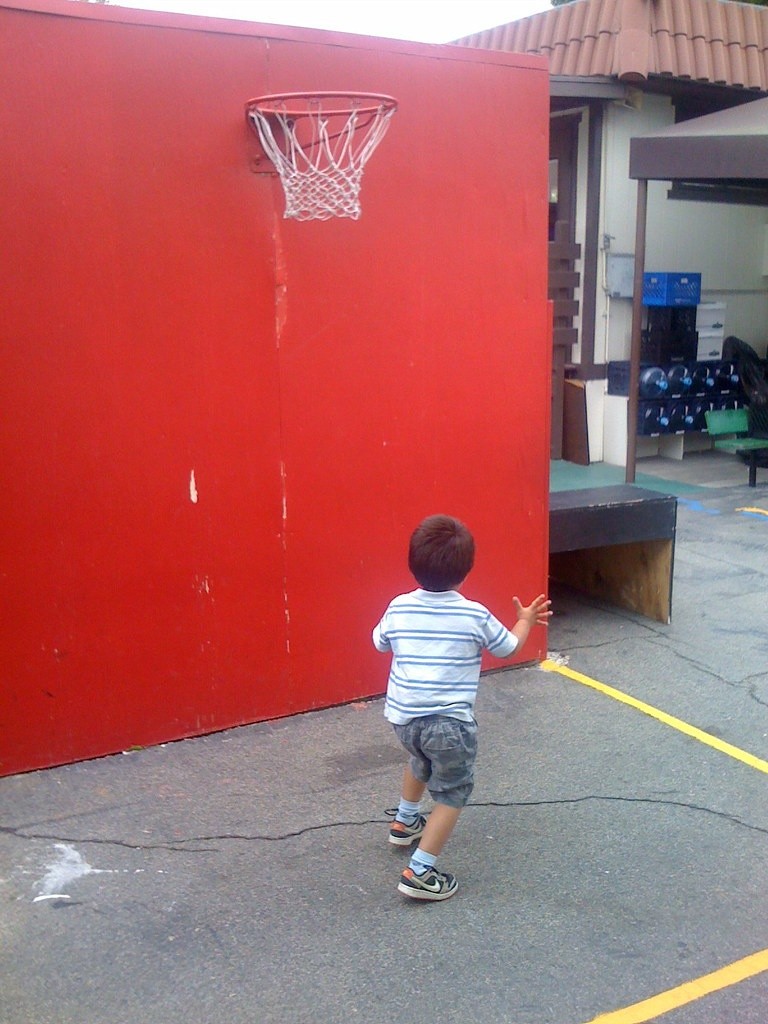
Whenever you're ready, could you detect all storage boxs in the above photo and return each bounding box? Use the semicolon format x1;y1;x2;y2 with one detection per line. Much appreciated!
642;272;728;367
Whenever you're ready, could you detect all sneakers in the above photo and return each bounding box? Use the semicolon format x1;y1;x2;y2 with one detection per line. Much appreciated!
384;808;428;846
397;865;459;900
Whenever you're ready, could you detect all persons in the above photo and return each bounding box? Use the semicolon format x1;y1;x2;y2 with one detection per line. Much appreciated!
371;514;552;901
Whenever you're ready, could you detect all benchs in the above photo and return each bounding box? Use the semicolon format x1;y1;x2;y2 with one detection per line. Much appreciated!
705;408;768;488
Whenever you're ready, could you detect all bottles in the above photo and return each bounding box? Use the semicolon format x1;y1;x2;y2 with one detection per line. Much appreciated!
607;360;669;400
690;396;742;433
661;363;693;397
708;361;739;390
666;399;695;433
638;400;671;435
685;363;716;392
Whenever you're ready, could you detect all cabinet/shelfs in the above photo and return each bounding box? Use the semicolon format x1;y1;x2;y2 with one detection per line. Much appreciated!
603;391;737;467
